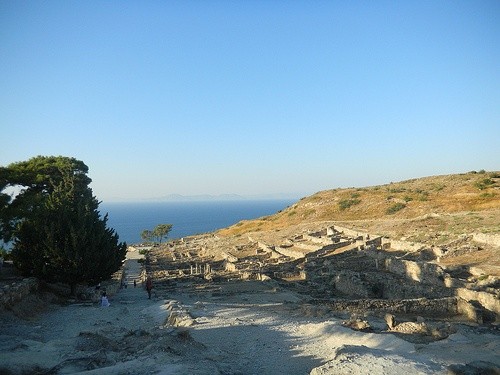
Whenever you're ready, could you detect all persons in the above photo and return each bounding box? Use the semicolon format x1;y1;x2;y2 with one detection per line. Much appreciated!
124;279;128;288
134;279;137;288
146;275;152;299
